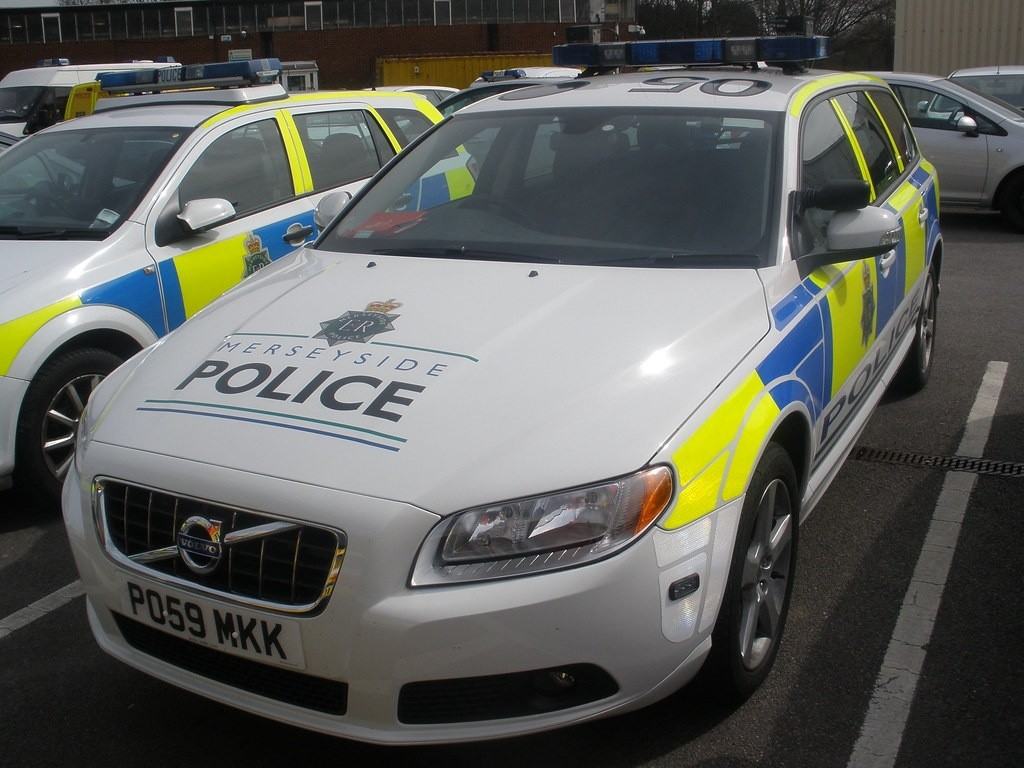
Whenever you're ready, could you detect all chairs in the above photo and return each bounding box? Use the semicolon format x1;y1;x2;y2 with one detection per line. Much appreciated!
317;132;365;184
668;149;747;263
143;146;170;190
539;140;643;245
736;128;771;199
633;120;722;200
217;136;274;203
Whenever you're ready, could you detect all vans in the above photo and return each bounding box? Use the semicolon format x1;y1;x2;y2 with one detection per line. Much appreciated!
0;55;183;154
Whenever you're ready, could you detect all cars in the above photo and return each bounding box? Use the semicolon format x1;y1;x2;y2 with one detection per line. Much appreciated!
853;71;1024;235
59;35;942;749
305;86;460;146
916;65;1024;120
0;58;490;524
463;67;583;108
